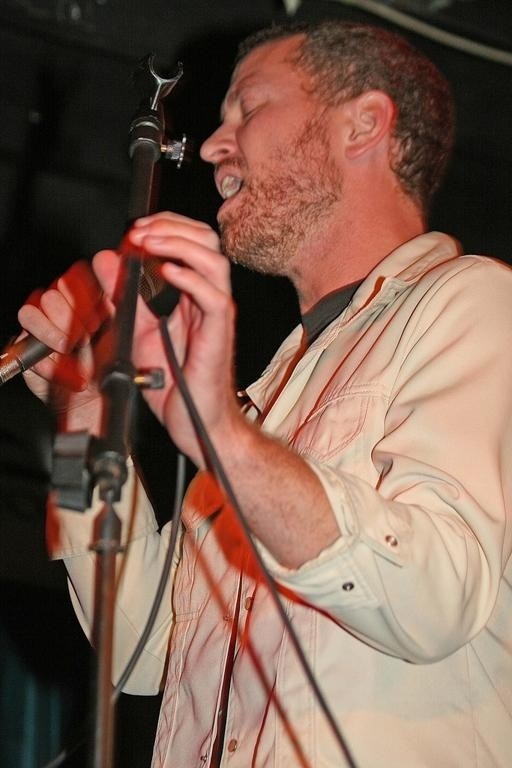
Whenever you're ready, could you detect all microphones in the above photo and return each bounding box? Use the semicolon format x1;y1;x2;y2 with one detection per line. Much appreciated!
1;260;176;388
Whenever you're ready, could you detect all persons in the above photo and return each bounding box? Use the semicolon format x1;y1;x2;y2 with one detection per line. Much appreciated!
13;13;512;768
